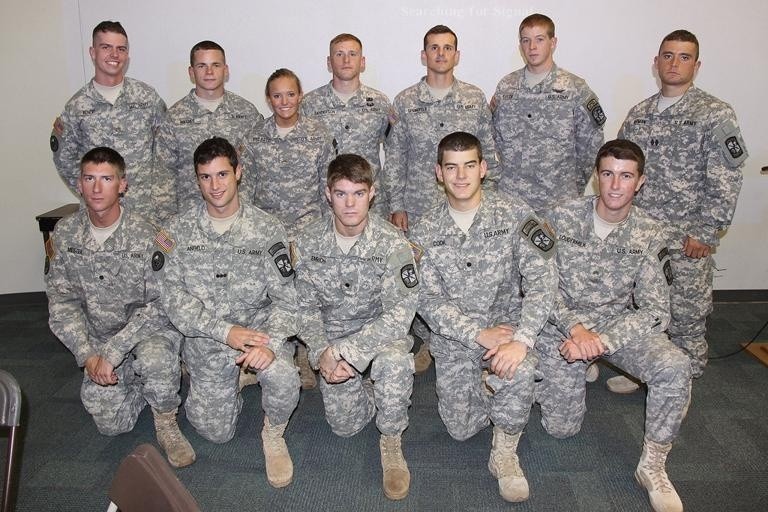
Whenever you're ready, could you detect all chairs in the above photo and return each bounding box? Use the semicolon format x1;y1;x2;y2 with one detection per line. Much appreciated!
0;367;26;511
105;442;201;512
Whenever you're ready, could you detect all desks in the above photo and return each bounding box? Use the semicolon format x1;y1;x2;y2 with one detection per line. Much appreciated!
35;202;81;254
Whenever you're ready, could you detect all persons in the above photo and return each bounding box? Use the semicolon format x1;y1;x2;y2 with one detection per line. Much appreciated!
42;146;200;471
532;137;698;510
151;39;268;395
294;149;421;501
382;23;501;381
604;25;751;397
237;66;338;395
48;18;184;380
152;137;301;490
407;128;562;504
489;11;610;387
294;33;408;234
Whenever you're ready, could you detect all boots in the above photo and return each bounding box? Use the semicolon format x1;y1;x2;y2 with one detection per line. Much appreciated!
295;344;318;390
150;406;197;469
487;426;531;503
260;414;295;489
379;433;411;502
632;436;685;512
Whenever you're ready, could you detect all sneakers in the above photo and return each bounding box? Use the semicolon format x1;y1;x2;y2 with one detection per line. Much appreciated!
413;343;429;376
238;367;259;392
584;363;600;384
606;374;644;395
